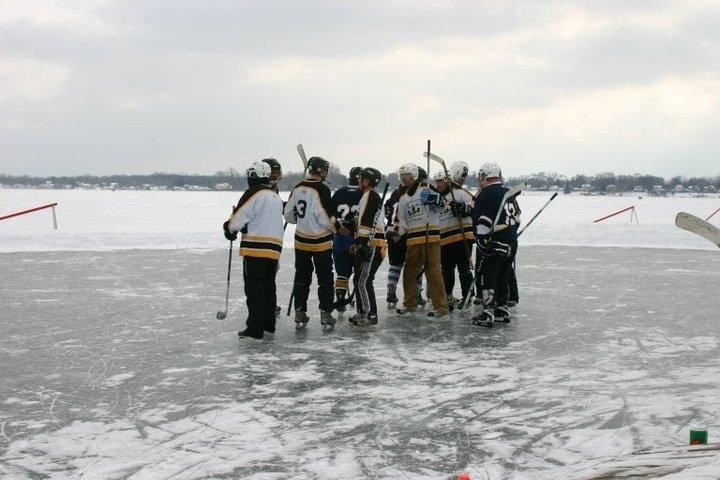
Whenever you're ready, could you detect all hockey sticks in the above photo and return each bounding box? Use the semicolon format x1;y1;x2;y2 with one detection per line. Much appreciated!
283;144;308;232
462;182;527;312
675;211;720;247
416;140;430;278
423;151;474;270
319;182;389;308
217;206;236;319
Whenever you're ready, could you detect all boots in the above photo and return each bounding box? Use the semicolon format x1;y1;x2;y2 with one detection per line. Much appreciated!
386;284;398;303
472;297;496;323
495;296;510;317
395;285;518;309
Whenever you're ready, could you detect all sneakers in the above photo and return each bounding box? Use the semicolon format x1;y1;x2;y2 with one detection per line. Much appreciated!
396;308;415;314
238;307;281;340
348;311;378;326
321;310;337;324
427;308;446;317
295;310;309;323
337;294;346;312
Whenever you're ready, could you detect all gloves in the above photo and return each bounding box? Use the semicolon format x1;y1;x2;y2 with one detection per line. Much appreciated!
449;200;473;217
420;188;443;208
223;220;239;241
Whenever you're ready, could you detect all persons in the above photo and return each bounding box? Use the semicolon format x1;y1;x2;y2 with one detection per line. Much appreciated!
222;157;522;339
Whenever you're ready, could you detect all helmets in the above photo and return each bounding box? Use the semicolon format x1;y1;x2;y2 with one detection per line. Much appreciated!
246;158;282;188
398;162;427;182
308;157;329;175
433;168;454;182
348;166;382;188
476;161;502;183
